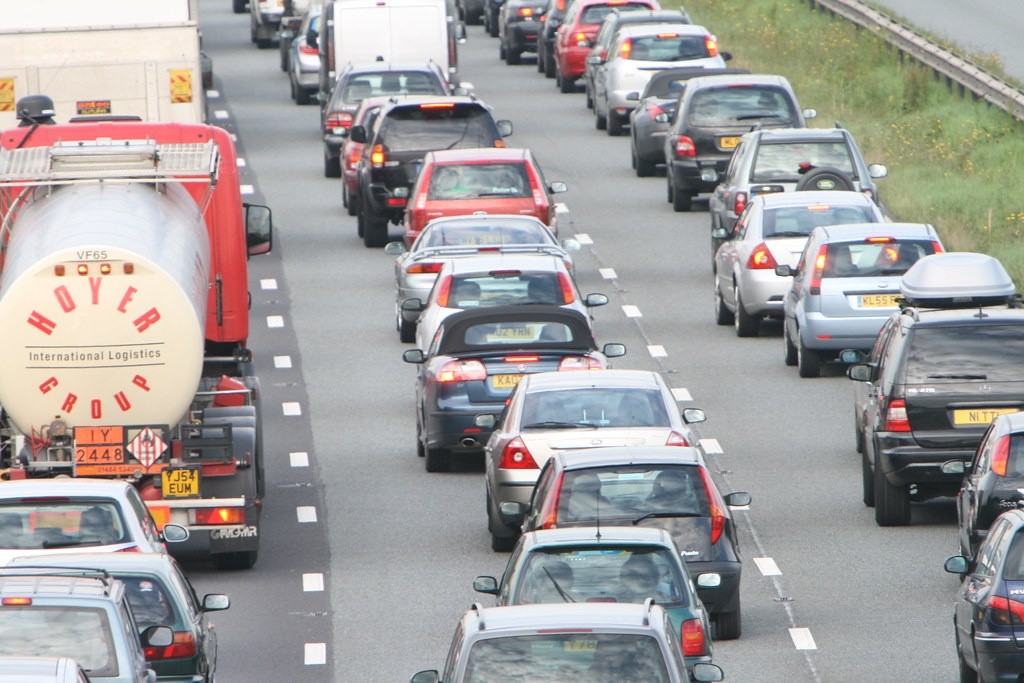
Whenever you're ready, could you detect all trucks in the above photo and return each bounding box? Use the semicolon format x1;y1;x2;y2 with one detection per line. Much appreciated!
316;0;456;122
1;94;275;575
1;0;214;125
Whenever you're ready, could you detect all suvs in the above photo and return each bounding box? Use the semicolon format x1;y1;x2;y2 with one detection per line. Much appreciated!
350;96;514;248
576;6;695;107
839;251;1024;526
709;119;888;244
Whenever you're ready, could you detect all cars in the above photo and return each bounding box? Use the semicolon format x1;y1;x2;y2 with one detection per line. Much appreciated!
454;0;661;93
586;25;734;135
0;564;157;683
774;223;947;374
943;500;1024;683
484;370;706;553
415;251;608;376
316;61;476;178
474;526;714;681
410;601;725;683
655;73;819;213
332;95;477;223
711;189;888;346
4;551;230;683
626;65;753;177
0;477;190;553
523;442;752;643
938;411;1024;584
402;305;627;474
392;146;569;249
385;214;582;346
0;656;92;682
232;0;323;105
